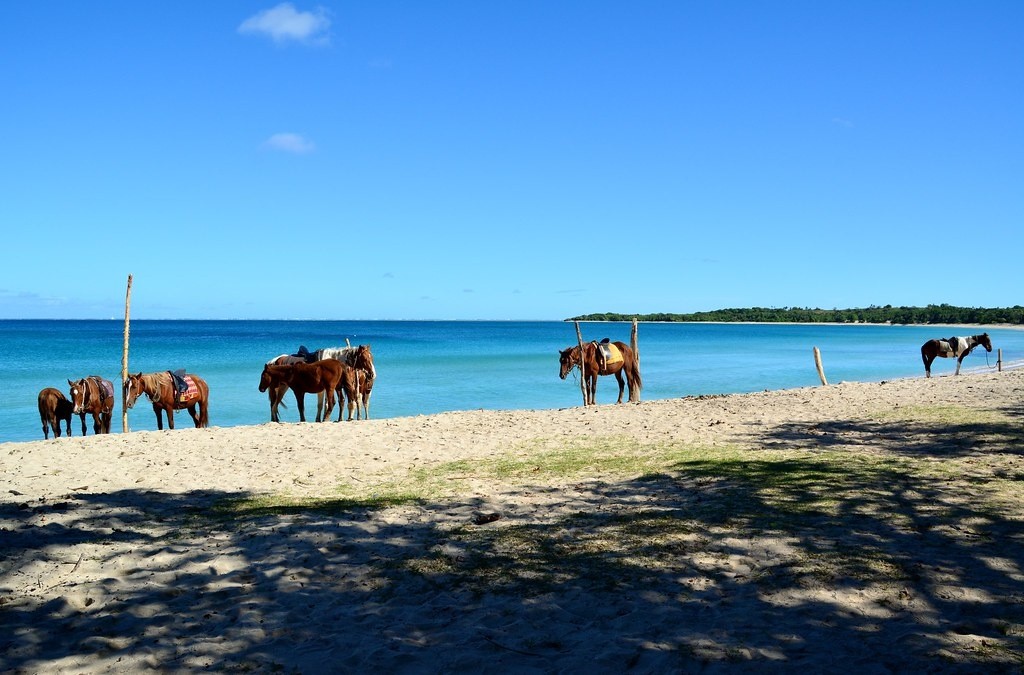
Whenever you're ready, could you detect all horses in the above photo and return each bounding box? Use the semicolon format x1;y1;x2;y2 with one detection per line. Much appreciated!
125;371;209;430
259;345;376;423
38;388;73;439
559;342;642;405
68;376;114;436
921;333;992;378
